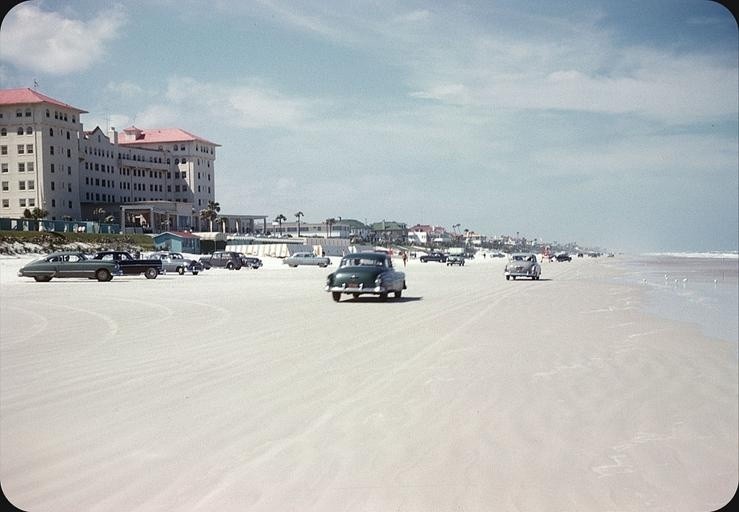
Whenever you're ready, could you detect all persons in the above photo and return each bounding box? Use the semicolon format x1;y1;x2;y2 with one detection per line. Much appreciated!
402;251;408;266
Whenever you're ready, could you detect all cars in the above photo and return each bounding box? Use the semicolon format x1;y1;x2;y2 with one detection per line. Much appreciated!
150;250;263;282
419;251;465;267
504;252;542;280
323;252;407;301
19;251;162;282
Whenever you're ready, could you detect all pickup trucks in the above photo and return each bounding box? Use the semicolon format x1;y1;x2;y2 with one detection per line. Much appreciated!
283;252;330;267
